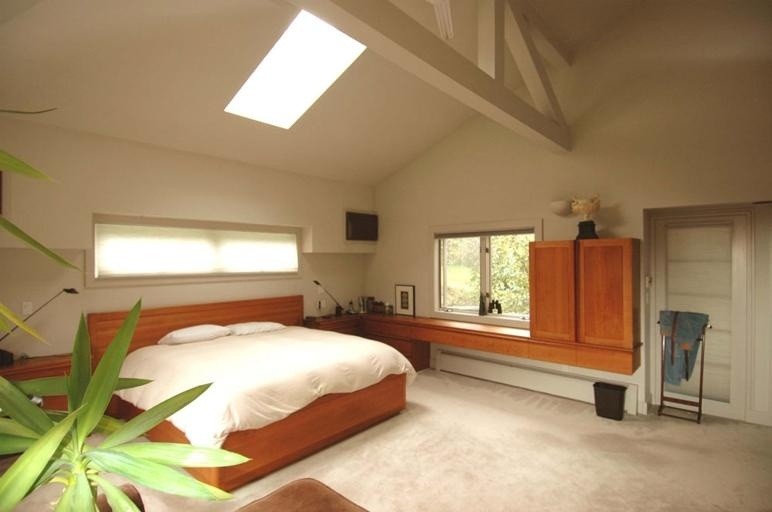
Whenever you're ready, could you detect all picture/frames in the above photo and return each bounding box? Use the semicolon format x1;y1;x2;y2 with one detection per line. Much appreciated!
394;284;416;316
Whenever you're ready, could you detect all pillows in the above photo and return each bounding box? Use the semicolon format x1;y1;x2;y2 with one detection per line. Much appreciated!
159;320;285;345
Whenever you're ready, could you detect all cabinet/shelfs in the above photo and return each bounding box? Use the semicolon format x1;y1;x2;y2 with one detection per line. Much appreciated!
529;237;642;349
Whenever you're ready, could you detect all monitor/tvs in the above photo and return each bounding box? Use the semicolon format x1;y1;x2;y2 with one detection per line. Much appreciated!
345;211;379;242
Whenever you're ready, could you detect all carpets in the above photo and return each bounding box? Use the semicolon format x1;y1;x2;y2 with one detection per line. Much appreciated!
235;478;367;512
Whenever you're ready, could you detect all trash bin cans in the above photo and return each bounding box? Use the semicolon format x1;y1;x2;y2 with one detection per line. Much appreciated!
593;382;628;421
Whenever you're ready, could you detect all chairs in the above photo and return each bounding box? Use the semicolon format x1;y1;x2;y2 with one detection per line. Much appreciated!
95;483;145;512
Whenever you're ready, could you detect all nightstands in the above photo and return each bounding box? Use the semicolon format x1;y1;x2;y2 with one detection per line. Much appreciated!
305;313;366;337
0;354;73;411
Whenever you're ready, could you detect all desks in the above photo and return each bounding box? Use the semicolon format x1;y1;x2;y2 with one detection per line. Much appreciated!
363;313;644;375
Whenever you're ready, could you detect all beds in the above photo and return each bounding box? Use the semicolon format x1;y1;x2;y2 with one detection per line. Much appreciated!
87;293;407;492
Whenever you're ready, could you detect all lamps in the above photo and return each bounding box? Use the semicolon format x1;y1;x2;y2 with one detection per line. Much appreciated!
312;279;345;316
0;288;79;365
